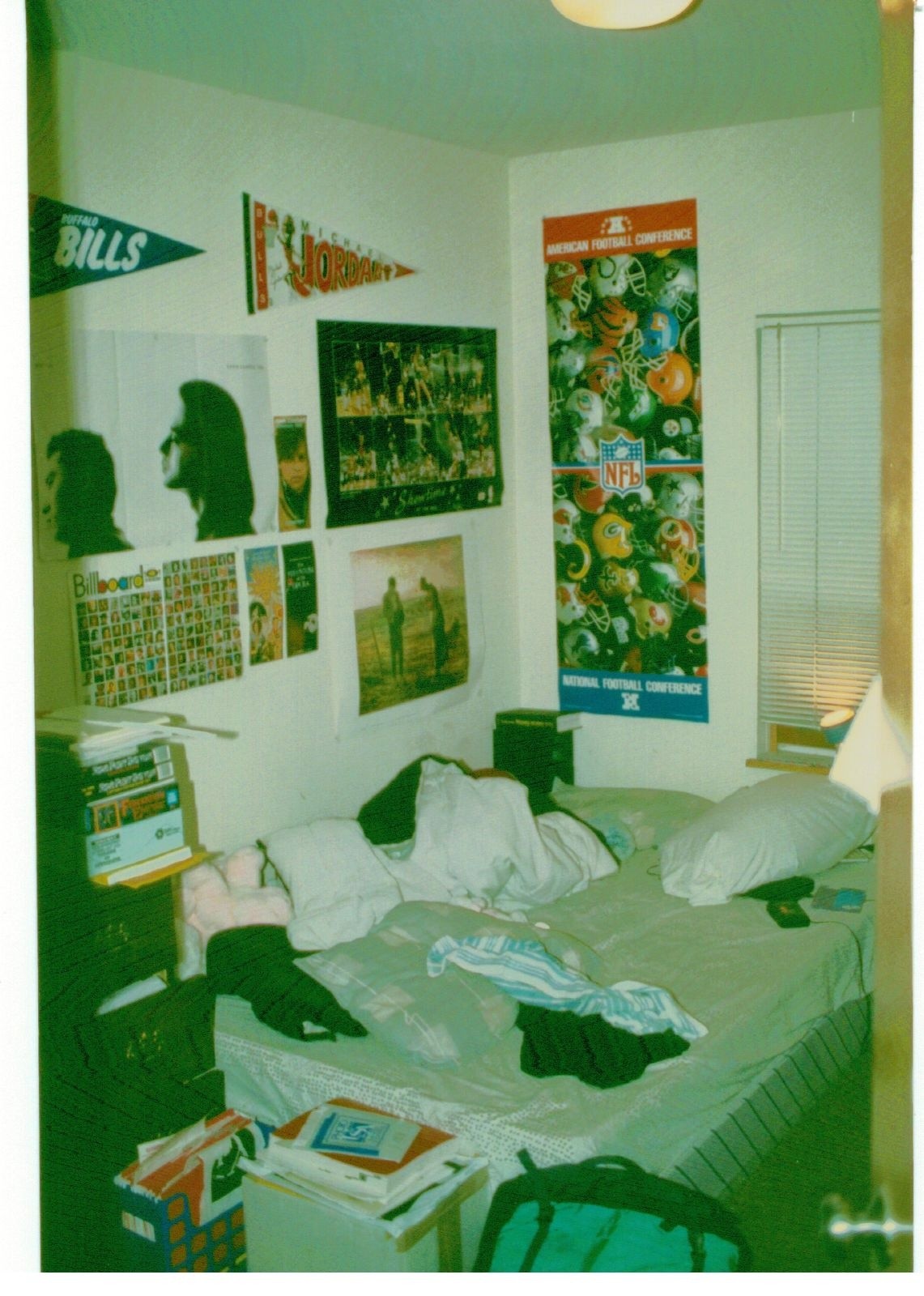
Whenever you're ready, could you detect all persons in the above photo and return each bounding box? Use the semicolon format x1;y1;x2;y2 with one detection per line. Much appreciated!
381;577;409;680
334;339;497;497
417;575;452;678
273;419;316;534
66;549;245;708
158;378;258;541
38;428;133;558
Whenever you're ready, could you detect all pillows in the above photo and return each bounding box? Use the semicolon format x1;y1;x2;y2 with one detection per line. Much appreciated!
295;898;602;1077
658;771;877;908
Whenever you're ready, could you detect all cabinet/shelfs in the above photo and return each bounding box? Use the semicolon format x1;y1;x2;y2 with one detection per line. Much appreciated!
40;869;225;1272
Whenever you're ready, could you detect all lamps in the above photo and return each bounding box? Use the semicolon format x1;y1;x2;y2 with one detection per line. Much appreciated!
819;706;854;748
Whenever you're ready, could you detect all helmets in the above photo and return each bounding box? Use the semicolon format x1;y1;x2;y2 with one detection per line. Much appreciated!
546;247;708;680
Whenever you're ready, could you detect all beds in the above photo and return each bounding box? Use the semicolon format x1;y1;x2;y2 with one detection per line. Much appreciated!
176;778;880;1219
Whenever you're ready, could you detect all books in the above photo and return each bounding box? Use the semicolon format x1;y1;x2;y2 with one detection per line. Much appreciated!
114;1092;494;1274
36;700;192;890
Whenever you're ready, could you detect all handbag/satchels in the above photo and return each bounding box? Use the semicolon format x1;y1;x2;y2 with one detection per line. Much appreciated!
472;1148;751;1272
